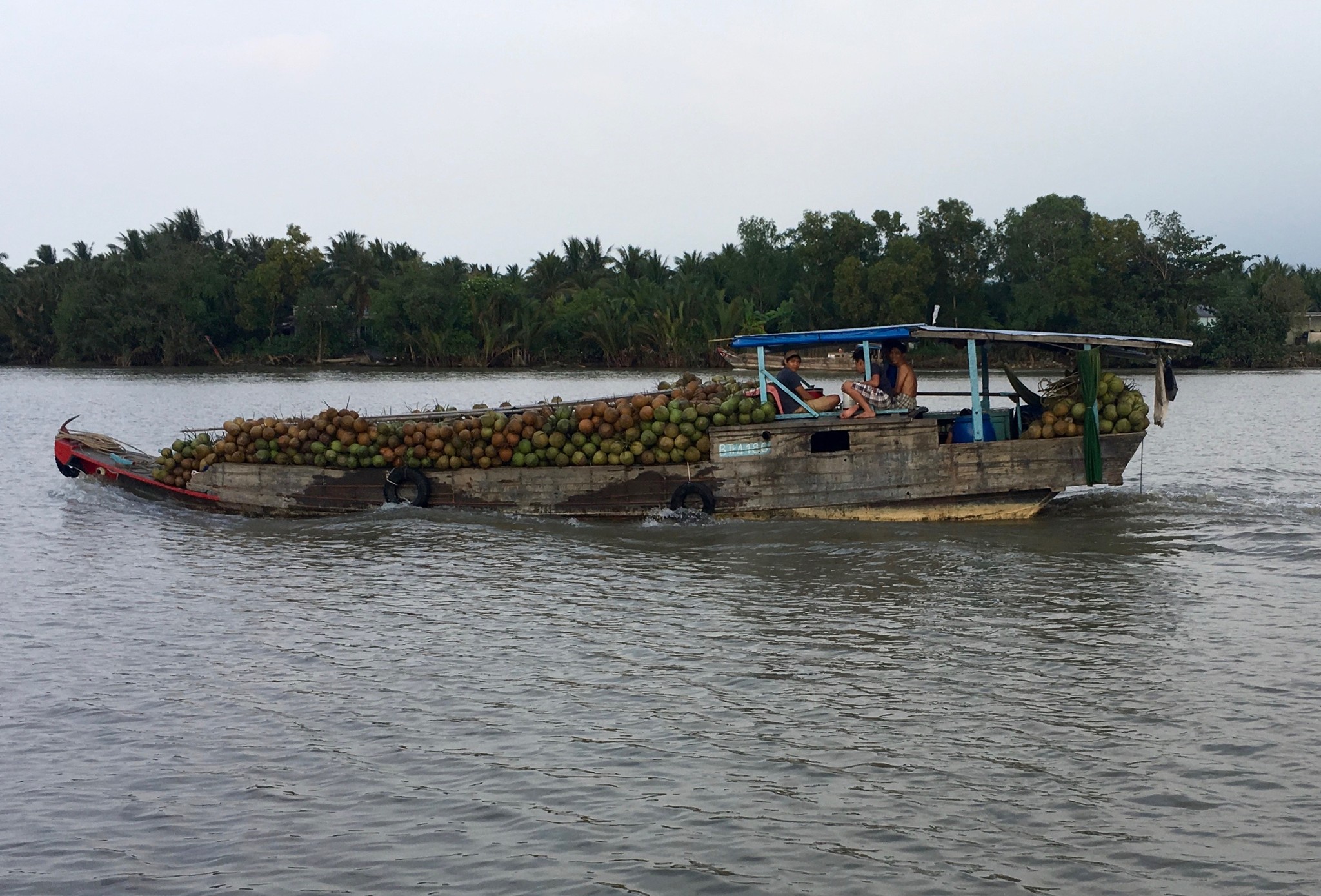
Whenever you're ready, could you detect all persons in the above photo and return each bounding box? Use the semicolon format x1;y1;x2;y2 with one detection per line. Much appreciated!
945;409;973;445
839;343;917;419
772;349;841;414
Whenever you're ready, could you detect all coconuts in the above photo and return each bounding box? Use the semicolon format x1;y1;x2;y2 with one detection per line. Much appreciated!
1027;373;1151;438
154;374;774;487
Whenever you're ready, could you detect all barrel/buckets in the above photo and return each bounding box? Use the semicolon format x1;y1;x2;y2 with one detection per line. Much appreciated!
953;413;996;443
842;390;856;410
805;388;823;399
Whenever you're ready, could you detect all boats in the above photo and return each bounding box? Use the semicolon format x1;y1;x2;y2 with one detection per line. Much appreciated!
716;342;883;372
356;359;397;367
54;323;1195;523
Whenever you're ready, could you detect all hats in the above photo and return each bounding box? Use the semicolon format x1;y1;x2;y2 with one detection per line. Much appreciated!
783;349;801;366
